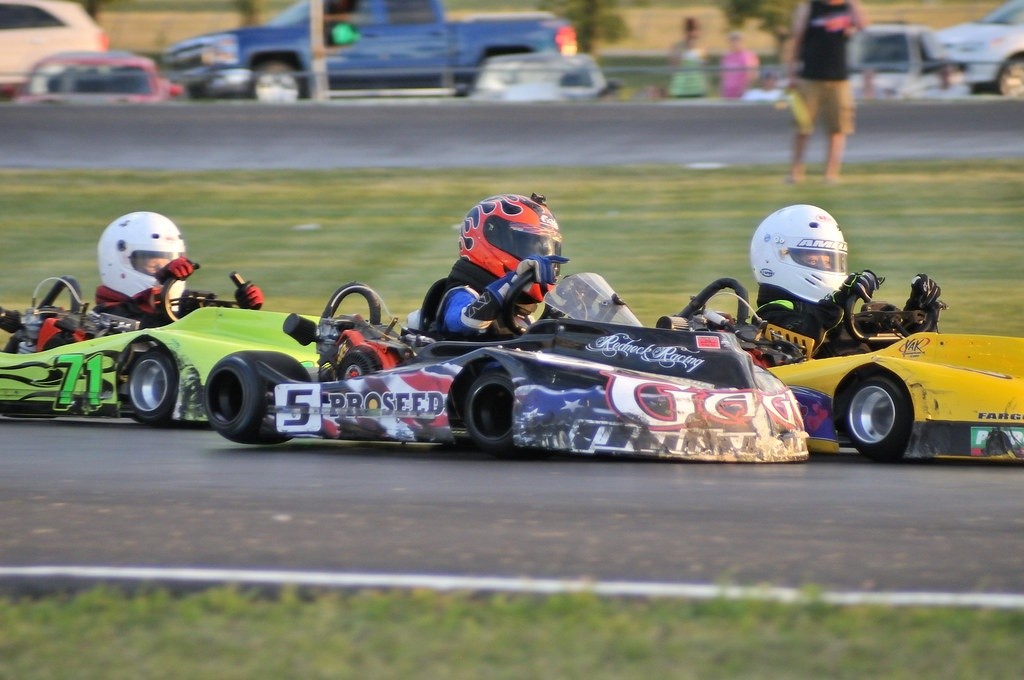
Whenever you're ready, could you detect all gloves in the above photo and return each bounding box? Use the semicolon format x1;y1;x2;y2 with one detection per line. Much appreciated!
155;257;200;285
903;274;942;311
484;253;571;308
823;269;885;310
234;281;263;310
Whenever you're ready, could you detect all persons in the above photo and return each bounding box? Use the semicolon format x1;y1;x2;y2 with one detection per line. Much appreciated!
421;192;599;364
740;72;787;101
750;204;941;361
91;209;264;334
667;17;712;100
719;28;760;98
776;0;867;188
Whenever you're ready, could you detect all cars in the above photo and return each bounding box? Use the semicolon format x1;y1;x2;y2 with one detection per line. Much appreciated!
0;0;111;101
931;0;1024;97
9;50;185;104
736;22;974;100
450;52;626;102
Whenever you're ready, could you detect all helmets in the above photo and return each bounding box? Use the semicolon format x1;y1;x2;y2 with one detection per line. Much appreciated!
750;204;848;308
459;194;562;304
97;211;186;300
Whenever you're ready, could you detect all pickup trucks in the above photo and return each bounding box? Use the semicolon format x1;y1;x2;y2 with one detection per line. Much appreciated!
159;0;579;105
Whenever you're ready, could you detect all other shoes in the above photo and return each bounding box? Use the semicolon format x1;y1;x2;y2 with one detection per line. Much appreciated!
825;166;839;185
789;163;806;185
